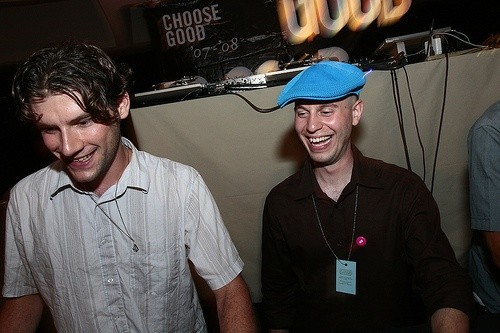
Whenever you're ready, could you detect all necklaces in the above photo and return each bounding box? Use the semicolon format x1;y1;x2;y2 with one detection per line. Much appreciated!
312;185;358;295
76;150;139;252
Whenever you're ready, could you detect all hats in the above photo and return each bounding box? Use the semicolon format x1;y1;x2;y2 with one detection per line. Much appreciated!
278;61;366;109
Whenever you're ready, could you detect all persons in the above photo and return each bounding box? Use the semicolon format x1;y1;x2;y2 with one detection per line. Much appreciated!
458;100;500;333
259;61;471;333
0;39;257;333
